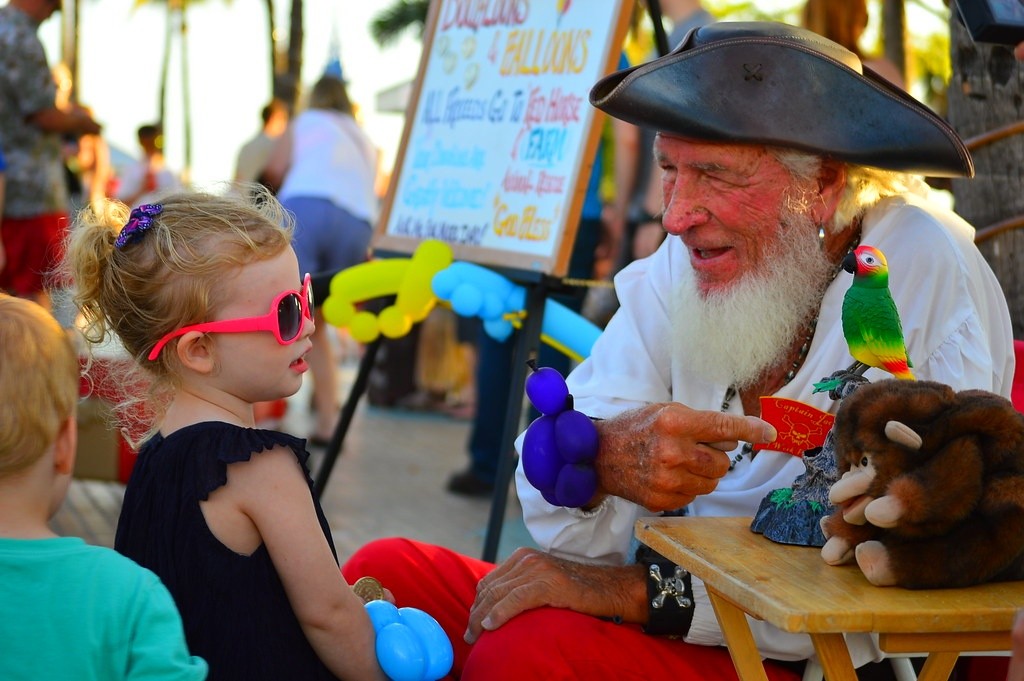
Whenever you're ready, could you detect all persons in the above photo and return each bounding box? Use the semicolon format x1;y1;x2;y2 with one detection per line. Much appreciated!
584;1;717;331
118;125;182;214
945;0;1024;97
341;18;1015;681
234;102;288;196
46;67;109;213
445;53;638;497
270;76;383;448
0;0;102;299
0;294;208;681
803;0;901;91
63;194;390;681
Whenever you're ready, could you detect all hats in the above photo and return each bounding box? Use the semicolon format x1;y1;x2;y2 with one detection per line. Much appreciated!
588;21;974;178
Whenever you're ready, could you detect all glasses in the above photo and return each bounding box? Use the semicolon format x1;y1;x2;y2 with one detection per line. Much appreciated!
148;275;317;359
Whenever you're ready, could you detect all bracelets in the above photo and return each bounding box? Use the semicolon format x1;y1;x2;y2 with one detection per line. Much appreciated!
626;208;663;230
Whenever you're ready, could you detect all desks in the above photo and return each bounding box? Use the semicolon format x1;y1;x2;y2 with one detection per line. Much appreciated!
634;516;1024;681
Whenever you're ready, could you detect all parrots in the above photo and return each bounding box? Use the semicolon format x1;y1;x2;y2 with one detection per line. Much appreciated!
813;246;917;401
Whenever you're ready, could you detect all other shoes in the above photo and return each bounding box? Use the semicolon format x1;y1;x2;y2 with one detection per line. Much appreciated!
307;430;341;453
310;396;345;414
444;460;511;501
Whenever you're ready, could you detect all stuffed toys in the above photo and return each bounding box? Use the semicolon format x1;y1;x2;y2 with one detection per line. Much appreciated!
821;379;1024;589
